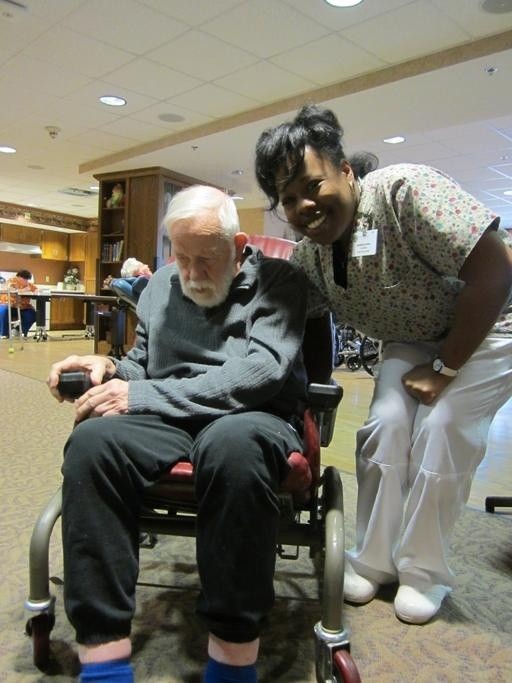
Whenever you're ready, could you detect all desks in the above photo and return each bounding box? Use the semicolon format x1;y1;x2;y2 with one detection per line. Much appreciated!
18;291;128;362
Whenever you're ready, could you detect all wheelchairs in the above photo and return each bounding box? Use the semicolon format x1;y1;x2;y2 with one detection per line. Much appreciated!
25;256;361;682
346;326;379;377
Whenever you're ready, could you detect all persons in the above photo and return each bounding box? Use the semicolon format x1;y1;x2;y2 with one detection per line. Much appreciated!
45;185;308;683
0;270;38;339
254;98;511;625
102;240;124;261
121;257;152;282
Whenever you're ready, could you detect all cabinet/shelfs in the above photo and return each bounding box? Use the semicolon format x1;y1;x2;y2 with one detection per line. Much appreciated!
93;169;130;348
131;166;200;346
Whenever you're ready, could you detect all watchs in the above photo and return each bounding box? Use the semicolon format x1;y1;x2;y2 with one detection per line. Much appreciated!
429;353;461;378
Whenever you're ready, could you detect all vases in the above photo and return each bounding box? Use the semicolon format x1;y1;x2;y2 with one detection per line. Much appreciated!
66;284;76;290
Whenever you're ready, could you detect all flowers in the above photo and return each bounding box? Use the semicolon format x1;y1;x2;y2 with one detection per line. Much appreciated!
63;262;81;284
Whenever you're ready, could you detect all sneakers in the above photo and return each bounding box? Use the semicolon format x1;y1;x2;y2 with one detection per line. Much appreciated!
1;336;8;339
343;566;378;604
11;328;19;336
393;581;443;624
